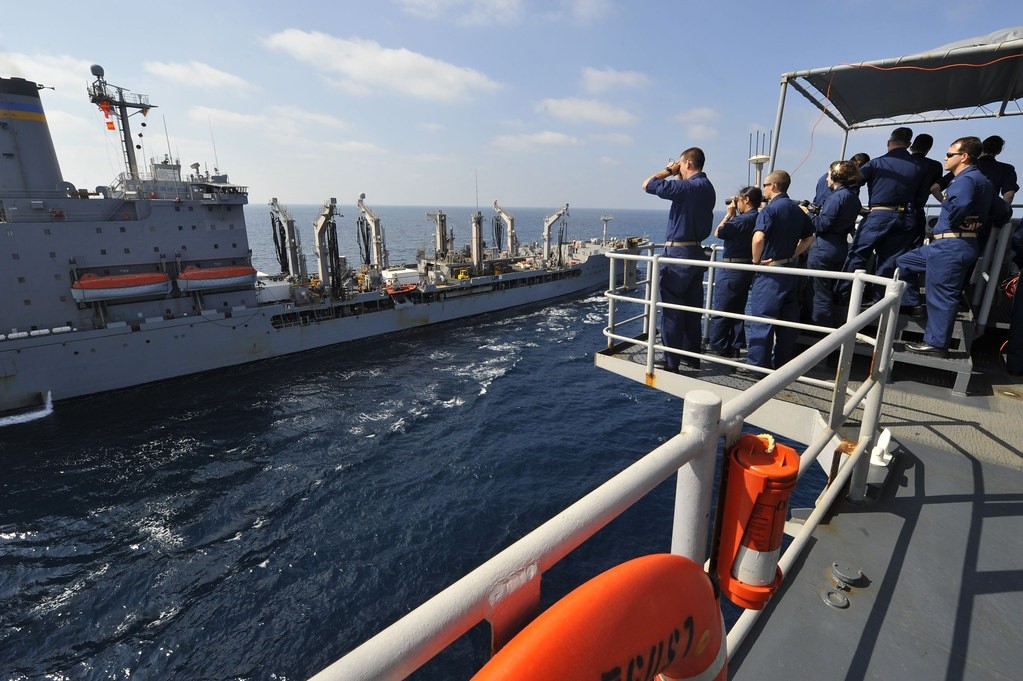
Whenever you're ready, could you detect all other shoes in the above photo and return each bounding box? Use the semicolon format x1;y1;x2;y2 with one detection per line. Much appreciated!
705;346;740;358
680;358;700;369
737;367;762;380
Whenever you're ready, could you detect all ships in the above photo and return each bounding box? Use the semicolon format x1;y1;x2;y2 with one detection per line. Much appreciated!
0;64;650;412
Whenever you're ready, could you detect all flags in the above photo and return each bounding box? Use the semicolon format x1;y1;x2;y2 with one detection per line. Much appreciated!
100;101;110;118
106;122;115;130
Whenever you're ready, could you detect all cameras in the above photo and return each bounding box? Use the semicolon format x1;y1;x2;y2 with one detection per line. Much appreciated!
964;216;982;228
726;197;738;207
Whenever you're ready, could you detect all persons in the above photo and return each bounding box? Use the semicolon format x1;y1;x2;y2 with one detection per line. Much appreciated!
643;147;716;373
813;152;870;237
799;160;862;327
930;135;1019;205
835;127;928;319
733;170;815;380
393;278;399;290
895;136;1012;358
703;186;762;358
1007;218;1023;376
910;134;943;250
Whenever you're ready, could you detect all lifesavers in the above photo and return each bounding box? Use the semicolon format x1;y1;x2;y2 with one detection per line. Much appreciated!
471;554;731;681
120;212;131;221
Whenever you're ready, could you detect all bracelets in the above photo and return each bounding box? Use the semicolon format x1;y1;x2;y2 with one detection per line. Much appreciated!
666;167;672;175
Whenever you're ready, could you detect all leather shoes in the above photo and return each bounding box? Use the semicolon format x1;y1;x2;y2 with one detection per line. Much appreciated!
900;305;927;318
904;341;947;357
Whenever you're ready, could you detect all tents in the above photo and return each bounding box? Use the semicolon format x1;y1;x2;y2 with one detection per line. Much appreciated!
767;24;1023;178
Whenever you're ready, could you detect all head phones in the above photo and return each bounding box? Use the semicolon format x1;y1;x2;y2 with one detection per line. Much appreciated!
830;162;842;183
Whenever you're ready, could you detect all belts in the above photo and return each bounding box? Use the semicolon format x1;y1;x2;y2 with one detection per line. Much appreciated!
664;241;701;246
722;258;752;262
764;258;793;266
870;206;905;211
933;233;978;239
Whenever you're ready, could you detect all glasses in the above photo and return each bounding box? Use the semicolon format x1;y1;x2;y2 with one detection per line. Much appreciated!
763;183;770;187
910;146;914;150
947;152;965;158
